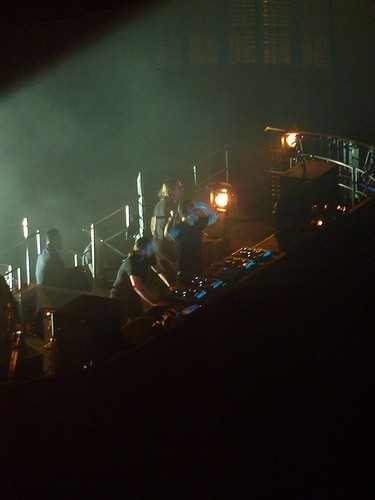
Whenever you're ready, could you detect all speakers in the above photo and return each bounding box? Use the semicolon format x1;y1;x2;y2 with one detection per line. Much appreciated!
276;208;312;250
281;159;338;220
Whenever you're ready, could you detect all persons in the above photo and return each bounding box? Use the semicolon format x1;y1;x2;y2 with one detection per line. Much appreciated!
110;237;173;347
269;198;294;234
0;275;21;382
35;229;76;291
163;198;212;294
81;233;109;289
150;177;185;290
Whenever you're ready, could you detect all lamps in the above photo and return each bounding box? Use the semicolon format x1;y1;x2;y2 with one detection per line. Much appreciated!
208;181;235;215
